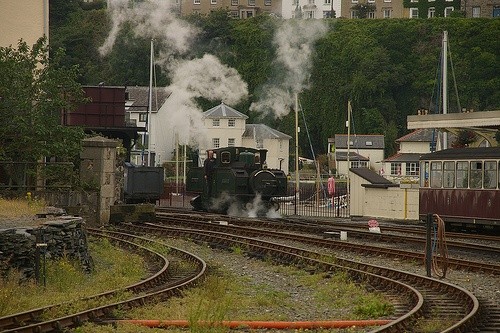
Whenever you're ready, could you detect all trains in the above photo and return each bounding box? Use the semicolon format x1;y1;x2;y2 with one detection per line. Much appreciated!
121;146;289;217
419;147;499;234
